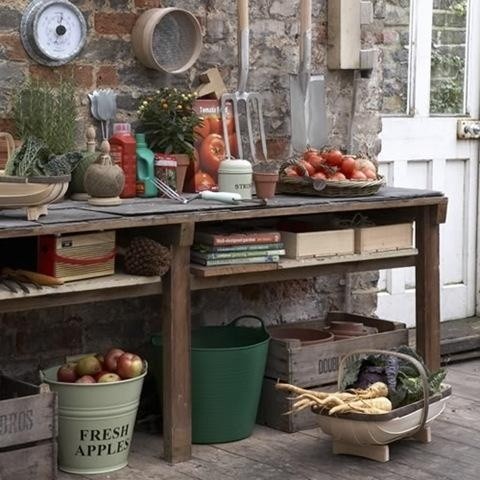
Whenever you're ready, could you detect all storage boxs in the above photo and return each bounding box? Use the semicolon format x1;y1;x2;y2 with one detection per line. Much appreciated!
255;378;339;434
264;311;408;388
0;376;59;449
0;441;59;479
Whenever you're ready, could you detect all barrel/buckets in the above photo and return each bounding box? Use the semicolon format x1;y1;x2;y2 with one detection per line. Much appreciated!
147;313;273;447
36;352;147;473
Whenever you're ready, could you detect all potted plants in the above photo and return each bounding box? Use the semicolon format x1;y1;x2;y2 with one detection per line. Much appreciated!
253;160;278;198
132;87;205;195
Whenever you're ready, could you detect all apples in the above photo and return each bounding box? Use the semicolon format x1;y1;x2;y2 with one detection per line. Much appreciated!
57;346;144;384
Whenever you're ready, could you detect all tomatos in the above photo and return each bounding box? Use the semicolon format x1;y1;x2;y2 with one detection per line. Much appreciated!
284;144;378;181
193;112;237;192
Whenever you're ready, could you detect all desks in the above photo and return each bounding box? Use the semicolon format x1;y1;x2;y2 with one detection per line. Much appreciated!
0;184;448;464
